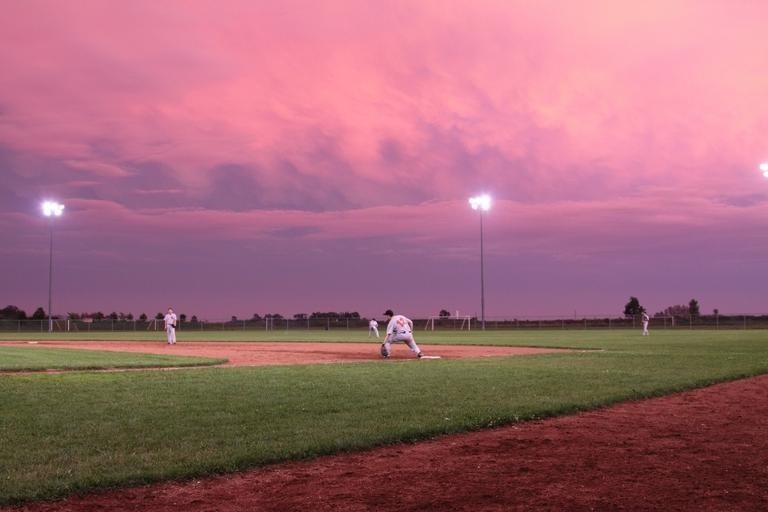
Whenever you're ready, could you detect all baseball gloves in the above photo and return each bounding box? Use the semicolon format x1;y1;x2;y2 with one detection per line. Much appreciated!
381;344;388;356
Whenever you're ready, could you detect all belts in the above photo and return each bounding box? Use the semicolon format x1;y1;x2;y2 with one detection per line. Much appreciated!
401;331;411;333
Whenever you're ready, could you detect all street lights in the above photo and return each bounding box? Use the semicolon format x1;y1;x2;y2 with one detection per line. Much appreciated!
471;193;492;330
44;200;64;331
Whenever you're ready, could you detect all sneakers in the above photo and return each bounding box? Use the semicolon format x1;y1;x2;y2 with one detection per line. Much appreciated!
417;353;423;360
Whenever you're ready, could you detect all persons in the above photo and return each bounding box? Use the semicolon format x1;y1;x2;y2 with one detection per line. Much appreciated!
380;309;423;359
368;318;380;338
641;312;650;337
163;307;177;344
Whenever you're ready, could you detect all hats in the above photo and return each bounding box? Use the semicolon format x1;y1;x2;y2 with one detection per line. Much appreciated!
383;310;392;315
